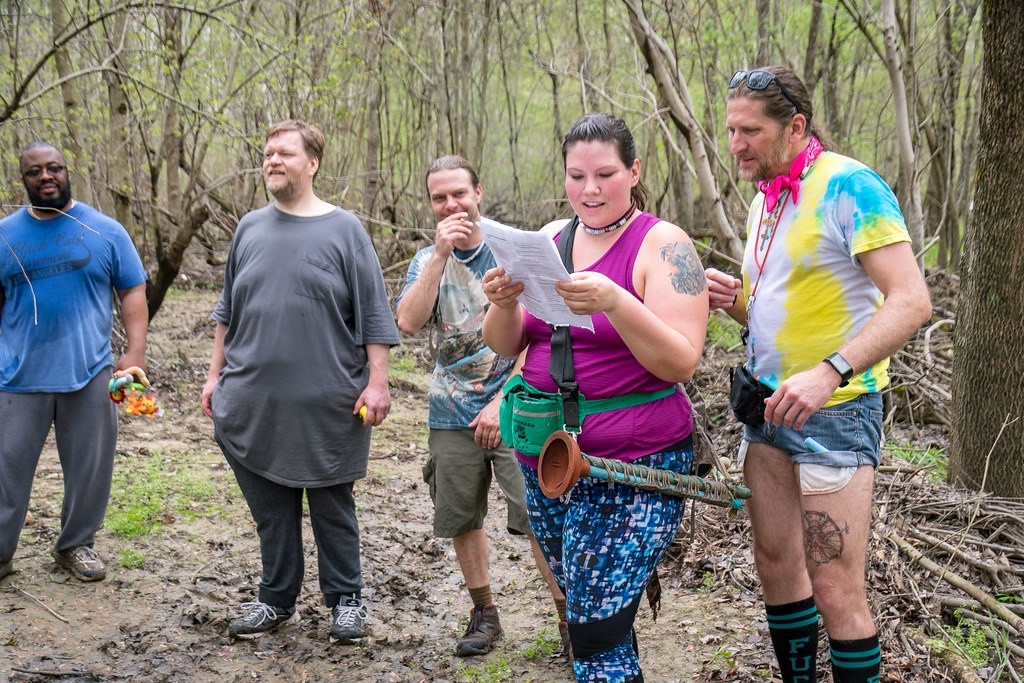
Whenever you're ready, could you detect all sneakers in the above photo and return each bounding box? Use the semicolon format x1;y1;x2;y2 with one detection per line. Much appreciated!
457;603;504;657
330;587;367;644
0;561;13;580
559;621;575;669
55;546;105;582
228;596;301;639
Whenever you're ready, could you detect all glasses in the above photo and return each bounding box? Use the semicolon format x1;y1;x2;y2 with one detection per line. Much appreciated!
728;70;802;116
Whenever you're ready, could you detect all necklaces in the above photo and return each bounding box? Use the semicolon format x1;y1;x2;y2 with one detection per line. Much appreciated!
448;242;483;265
574;196;636;236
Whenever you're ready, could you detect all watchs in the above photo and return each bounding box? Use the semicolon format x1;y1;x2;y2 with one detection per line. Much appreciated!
822;352;854;387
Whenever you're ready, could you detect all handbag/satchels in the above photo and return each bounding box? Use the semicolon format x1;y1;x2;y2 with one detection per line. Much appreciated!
500;375;678;458
731;364;776;426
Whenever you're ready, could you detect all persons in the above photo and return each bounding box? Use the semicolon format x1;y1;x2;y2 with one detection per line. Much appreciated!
198;118;402;643
480;110;710;683
705;58;936;683
0;141;162;588
394;153;588;659
